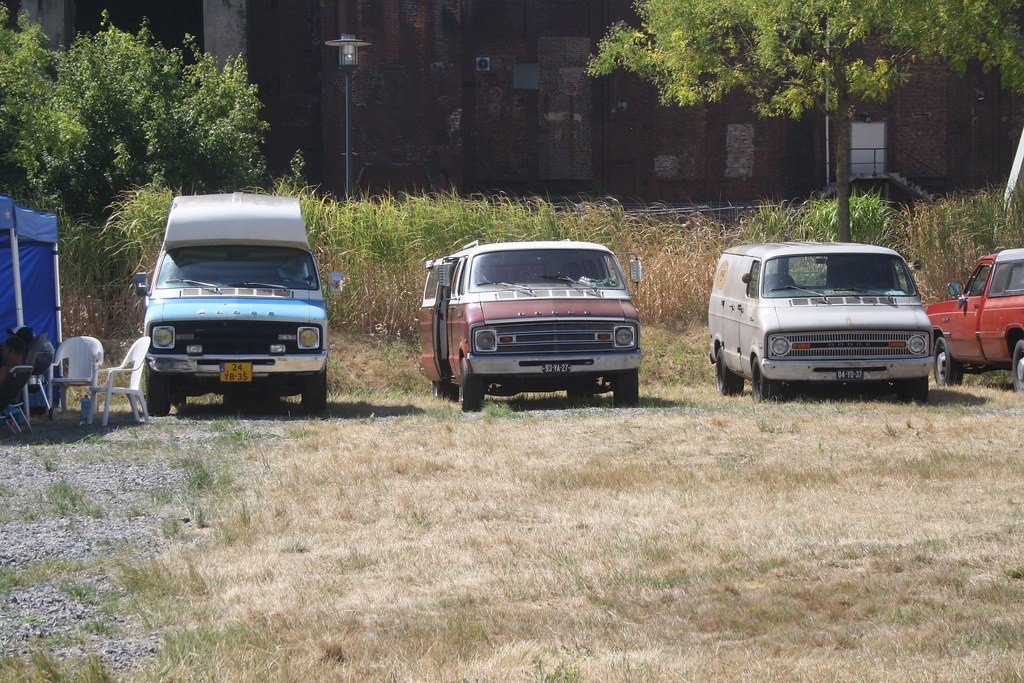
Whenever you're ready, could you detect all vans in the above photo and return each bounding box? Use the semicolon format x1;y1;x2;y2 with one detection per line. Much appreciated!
707;241;935;402
417;241;643;412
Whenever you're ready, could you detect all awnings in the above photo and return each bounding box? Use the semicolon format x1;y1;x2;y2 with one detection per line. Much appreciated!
0;197;62;422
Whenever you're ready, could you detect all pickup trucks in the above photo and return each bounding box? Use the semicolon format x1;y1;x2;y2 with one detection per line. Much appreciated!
923;248;1024;392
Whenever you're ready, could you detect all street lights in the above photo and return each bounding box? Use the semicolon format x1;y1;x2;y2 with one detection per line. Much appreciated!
325;32;373;202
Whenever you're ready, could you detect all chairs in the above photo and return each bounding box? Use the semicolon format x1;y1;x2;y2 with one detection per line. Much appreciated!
765;273;796;290
87;336;151;426
0;365;34;434
49;336;104;414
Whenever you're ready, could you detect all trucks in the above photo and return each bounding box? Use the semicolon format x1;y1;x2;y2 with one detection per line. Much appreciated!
134;192;344;419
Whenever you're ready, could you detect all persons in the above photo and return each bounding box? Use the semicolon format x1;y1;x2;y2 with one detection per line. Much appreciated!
0;334;28;413
16;327;35;343
